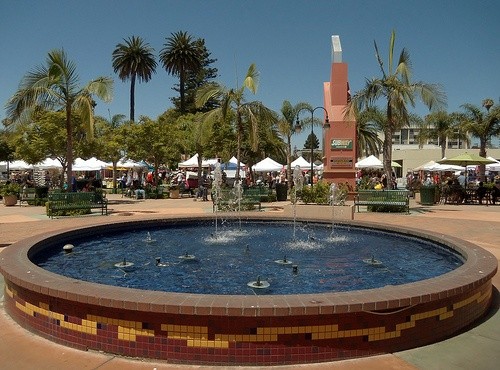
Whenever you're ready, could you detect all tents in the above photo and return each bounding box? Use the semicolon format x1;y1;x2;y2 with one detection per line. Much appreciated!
0;156;500;180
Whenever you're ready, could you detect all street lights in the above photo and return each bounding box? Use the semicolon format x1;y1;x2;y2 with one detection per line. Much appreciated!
295;107;331;185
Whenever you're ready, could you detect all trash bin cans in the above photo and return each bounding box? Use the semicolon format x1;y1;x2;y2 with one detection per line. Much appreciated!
275;182;288;201
419;185;436;205
34;185;49;206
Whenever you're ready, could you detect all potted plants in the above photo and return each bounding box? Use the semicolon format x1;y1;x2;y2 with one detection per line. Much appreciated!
295;178;328;205
0;179;24;206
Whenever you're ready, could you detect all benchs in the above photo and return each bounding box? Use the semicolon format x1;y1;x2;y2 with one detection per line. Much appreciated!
210;188;262;211
355;190;410;215
47;191;109;220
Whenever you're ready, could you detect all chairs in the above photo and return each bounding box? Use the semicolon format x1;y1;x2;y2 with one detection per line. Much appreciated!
19;187;37;206
443;184;494;206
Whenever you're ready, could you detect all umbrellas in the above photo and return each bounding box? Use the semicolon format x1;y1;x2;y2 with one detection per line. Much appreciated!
438;153;498;201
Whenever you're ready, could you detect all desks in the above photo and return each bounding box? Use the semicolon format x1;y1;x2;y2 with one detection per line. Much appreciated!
463;187;494;205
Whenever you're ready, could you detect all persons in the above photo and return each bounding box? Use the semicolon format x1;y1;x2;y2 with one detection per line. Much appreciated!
0;171;500;205
